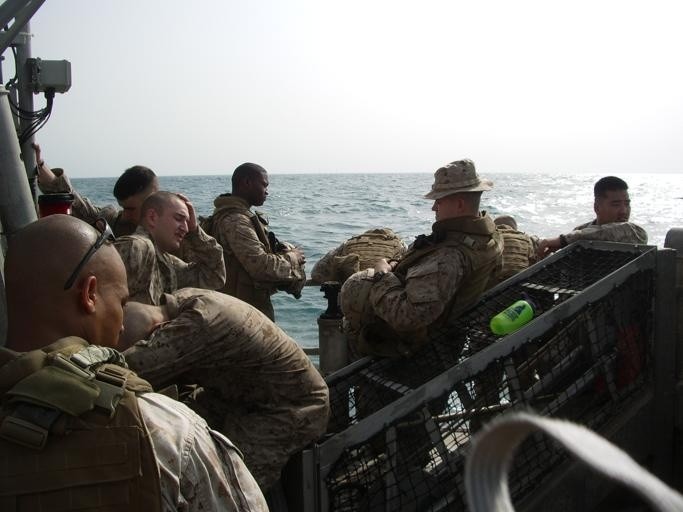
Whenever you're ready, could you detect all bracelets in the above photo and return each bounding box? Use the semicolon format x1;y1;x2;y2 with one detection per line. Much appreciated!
559;234;567;248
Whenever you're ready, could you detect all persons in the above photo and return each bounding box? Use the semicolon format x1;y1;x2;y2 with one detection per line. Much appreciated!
537;177;648;259
0;141;546;510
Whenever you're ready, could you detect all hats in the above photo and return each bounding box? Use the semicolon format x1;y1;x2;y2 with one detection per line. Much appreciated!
65;213;116;289
421;158;494;200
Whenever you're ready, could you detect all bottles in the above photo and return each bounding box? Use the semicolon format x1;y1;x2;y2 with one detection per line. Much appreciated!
490;298;536;338
